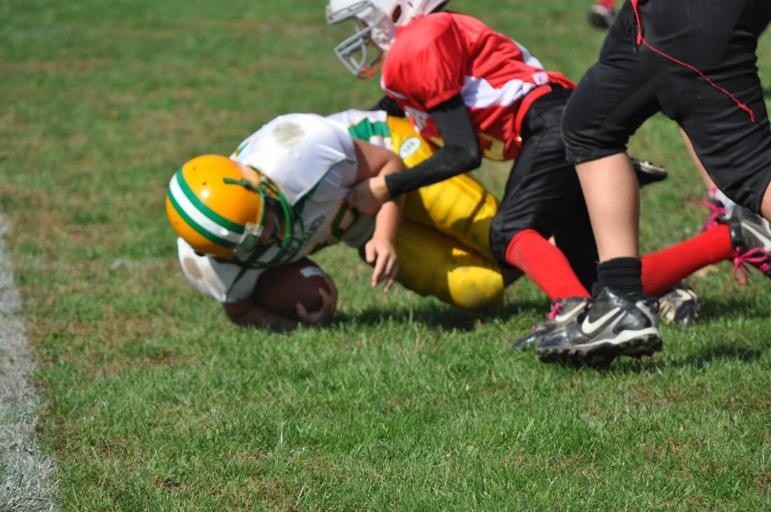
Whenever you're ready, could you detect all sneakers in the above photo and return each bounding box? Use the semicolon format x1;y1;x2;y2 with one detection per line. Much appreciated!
510;287;701;369
588;5;614;28
627;157;667;185
702;189;770;278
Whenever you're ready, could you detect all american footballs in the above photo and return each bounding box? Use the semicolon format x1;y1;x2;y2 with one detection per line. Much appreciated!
254;260;332;319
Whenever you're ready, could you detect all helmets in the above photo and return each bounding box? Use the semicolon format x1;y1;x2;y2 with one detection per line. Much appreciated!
163;152;305;271
324;0;450;81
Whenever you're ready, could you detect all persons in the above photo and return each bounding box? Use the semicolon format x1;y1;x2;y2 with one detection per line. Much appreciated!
510;2;771;368
164;110;668;330
326;1;769;350
